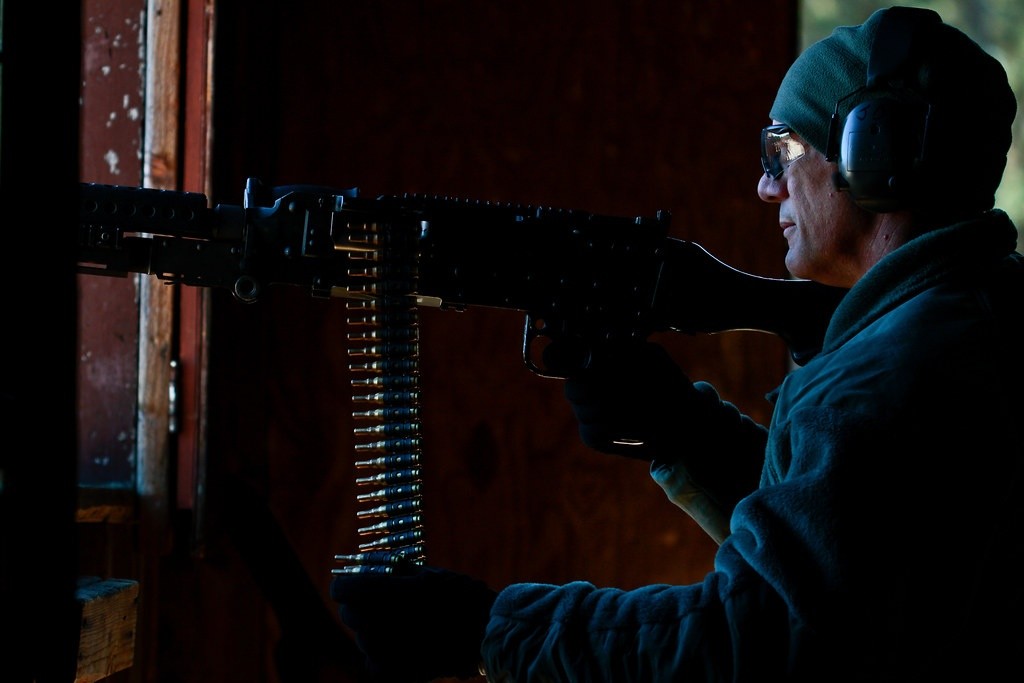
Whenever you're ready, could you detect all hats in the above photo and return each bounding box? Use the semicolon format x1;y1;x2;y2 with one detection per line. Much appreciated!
769;24;1018;212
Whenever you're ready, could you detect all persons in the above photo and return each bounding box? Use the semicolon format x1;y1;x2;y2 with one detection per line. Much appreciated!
330;6;1024;683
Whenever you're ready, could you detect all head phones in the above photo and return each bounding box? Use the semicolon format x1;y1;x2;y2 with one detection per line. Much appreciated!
835;4;942;212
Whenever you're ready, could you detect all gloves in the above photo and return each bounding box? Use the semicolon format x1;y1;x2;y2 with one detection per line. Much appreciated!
324;548;502;678
538;331;698;465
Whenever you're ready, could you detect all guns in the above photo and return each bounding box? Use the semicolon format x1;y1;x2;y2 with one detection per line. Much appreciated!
37;158;868;369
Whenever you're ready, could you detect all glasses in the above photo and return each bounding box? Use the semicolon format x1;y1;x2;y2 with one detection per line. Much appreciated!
759;123;807;187
